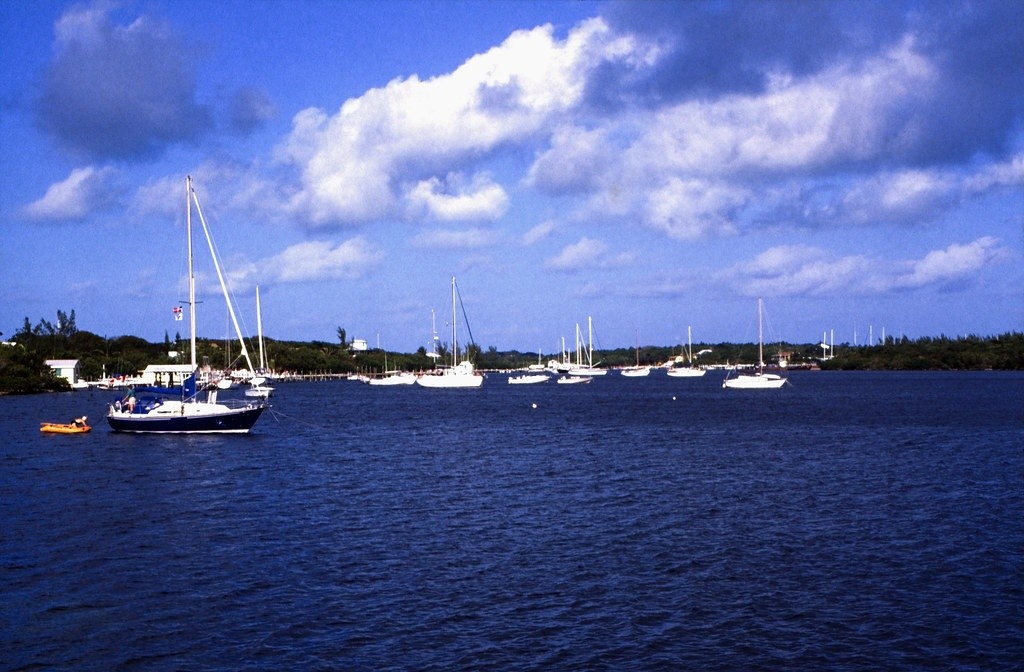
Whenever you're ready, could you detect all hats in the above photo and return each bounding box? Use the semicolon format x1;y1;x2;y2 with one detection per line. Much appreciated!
82;416;87;421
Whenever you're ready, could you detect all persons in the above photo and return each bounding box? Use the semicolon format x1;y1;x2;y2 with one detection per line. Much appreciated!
122;384;136;412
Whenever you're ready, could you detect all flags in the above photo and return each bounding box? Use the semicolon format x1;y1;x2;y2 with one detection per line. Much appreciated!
173;306;183;320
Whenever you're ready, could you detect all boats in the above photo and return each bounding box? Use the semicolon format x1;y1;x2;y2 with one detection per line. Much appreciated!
507;373;551;385
557;376;591;386
38;417;91;433
367;371;419;386
68;320;613;393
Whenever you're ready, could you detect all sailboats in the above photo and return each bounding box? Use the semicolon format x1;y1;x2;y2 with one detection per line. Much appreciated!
104;174;273;436
721;297;790;389
620;327;652;378
415;276;486;387
665;325;709;377
